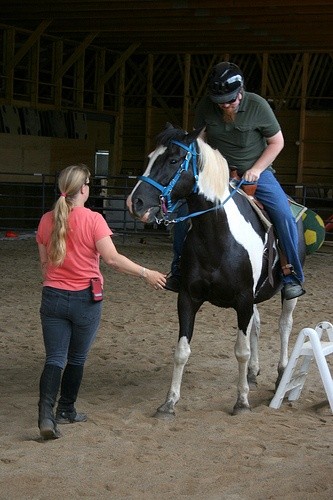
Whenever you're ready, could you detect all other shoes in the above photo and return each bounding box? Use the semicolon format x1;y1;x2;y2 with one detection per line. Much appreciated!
283;280;302;299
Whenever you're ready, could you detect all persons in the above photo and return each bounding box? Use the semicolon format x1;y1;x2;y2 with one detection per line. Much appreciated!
36;164;168;441
162;62;307;300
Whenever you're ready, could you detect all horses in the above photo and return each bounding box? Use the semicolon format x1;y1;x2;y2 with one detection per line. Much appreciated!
126;120;305;422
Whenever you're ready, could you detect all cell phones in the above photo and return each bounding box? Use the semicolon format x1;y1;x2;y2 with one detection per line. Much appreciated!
91;278;103;301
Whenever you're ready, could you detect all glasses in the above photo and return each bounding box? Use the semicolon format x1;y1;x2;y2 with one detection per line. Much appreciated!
218;93;239;104
84;182;91;187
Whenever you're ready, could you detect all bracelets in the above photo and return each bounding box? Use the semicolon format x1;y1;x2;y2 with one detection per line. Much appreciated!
140;267;145;280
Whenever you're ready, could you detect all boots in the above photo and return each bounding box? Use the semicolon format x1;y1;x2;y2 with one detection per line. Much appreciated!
37;364;63;440
54;362;85;423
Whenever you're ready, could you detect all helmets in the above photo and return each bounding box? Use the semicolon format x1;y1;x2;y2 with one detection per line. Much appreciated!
208;62;245;103
